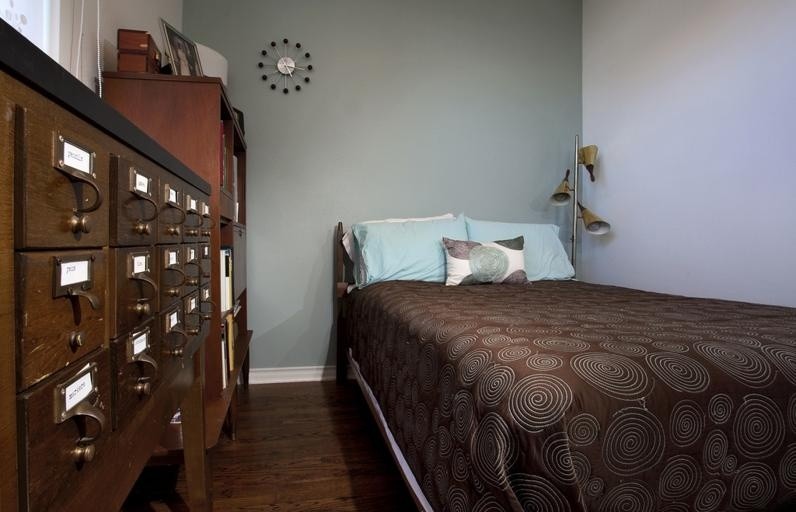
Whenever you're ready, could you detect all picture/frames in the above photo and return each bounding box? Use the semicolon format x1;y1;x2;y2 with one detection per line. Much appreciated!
158;16;204;76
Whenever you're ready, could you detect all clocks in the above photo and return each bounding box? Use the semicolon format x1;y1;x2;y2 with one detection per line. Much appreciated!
258;39;313;93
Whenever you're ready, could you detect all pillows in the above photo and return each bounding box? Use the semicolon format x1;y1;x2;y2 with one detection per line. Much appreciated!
339;212;578;288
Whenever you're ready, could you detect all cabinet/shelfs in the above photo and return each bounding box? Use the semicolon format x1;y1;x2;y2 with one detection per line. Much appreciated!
100;72;252;442
2;76;215;512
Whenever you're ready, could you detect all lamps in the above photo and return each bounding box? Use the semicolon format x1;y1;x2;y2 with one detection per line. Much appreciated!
546;134;611;275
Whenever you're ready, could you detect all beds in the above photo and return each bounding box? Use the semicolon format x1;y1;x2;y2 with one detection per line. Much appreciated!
330;218;796;511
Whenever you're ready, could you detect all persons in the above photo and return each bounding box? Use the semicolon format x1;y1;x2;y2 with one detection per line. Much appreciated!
176;38;198;77
